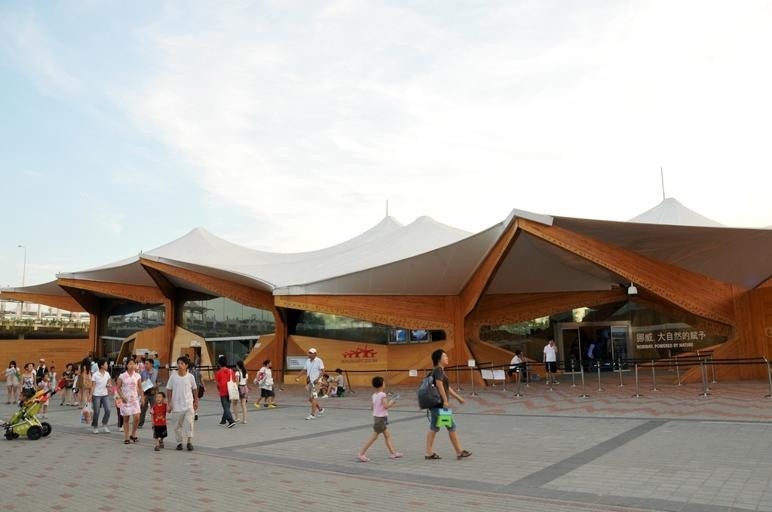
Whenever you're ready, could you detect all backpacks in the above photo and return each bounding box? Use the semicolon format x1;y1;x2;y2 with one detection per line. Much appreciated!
417;376;442;410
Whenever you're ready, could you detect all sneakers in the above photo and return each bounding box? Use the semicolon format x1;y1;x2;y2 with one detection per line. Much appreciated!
218;418;247;428
358;453;370;462
318;408;325;418
92;415;197;451
305;414;316;420
254;402;277;408
388;452;401;459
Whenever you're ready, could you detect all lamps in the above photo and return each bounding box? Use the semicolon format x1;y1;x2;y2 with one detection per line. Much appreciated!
623;282;641;297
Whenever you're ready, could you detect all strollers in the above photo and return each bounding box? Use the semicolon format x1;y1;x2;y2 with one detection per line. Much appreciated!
0;377;63;440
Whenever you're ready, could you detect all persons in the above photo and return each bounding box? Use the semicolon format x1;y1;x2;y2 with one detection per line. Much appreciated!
542;338;561;386
570;331;628;372
213;355;248;428
423;349;473;461
114;352;203;450
294;348;327;421
357;375;405;462
5;358;56;421
255;359;277;408
320;368;345;398
508;349;528;392
60;355;112;434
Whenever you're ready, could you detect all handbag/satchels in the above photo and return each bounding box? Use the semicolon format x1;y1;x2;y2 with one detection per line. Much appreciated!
227;381;239;400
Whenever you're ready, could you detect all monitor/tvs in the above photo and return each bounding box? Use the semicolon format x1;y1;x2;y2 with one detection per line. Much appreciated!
409;330;431;343
387;328;409;344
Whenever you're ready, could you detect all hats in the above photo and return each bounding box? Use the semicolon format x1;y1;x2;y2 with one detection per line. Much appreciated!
306;348;317;354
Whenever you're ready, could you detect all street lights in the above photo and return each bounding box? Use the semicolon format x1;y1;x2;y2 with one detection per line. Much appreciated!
16;243;26;288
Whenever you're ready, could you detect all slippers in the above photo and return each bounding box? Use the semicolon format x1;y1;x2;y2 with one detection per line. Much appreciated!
425;453;442;459
457;449;472;457
